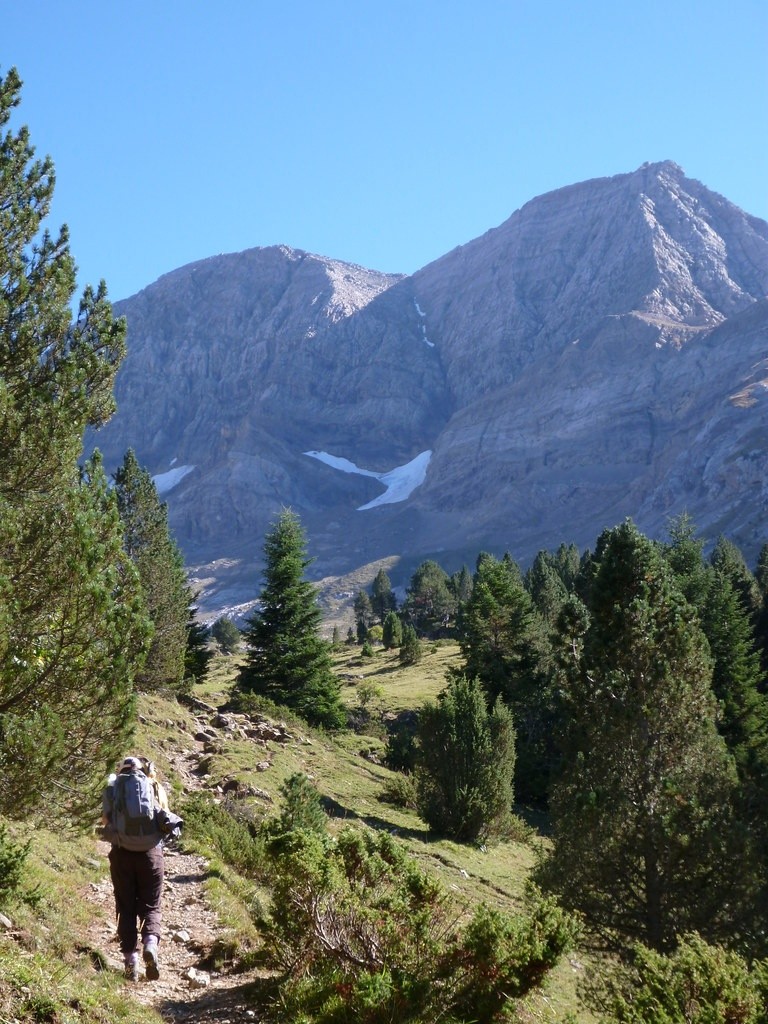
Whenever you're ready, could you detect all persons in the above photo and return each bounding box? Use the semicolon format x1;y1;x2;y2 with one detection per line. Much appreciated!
101;758;170;980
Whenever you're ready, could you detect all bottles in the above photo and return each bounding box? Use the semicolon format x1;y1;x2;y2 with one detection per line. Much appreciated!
107;773;116;787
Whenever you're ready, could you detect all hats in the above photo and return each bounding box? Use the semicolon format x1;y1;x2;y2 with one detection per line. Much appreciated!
123;757;143;769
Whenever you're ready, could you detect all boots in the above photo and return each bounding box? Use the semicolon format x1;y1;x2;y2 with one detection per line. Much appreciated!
123;952;138;982
143;935;160;981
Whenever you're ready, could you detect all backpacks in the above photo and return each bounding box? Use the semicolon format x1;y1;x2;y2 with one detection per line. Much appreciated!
103;770;170;852
